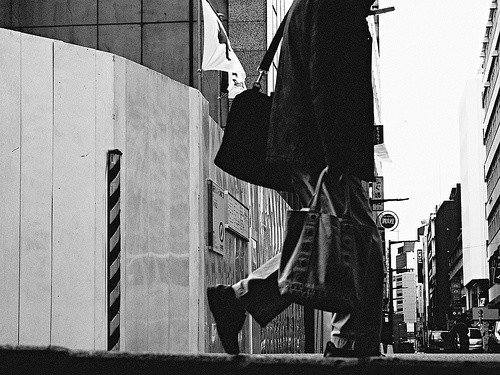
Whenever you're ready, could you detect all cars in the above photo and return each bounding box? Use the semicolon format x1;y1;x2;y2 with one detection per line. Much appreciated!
430;327;483;352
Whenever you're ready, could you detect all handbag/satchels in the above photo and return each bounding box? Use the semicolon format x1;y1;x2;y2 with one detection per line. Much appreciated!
214;89;295;193
278;165;374;314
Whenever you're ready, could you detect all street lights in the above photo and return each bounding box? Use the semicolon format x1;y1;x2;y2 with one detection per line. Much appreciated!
388;240;421;344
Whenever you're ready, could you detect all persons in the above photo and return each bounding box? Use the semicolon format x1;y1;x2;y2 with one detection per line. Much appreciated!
207;0;385;359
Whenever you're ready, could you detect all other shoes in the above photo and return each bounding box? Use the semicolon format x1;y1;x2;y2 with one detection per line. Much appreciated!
323;341;357;358
207;283;246;354
